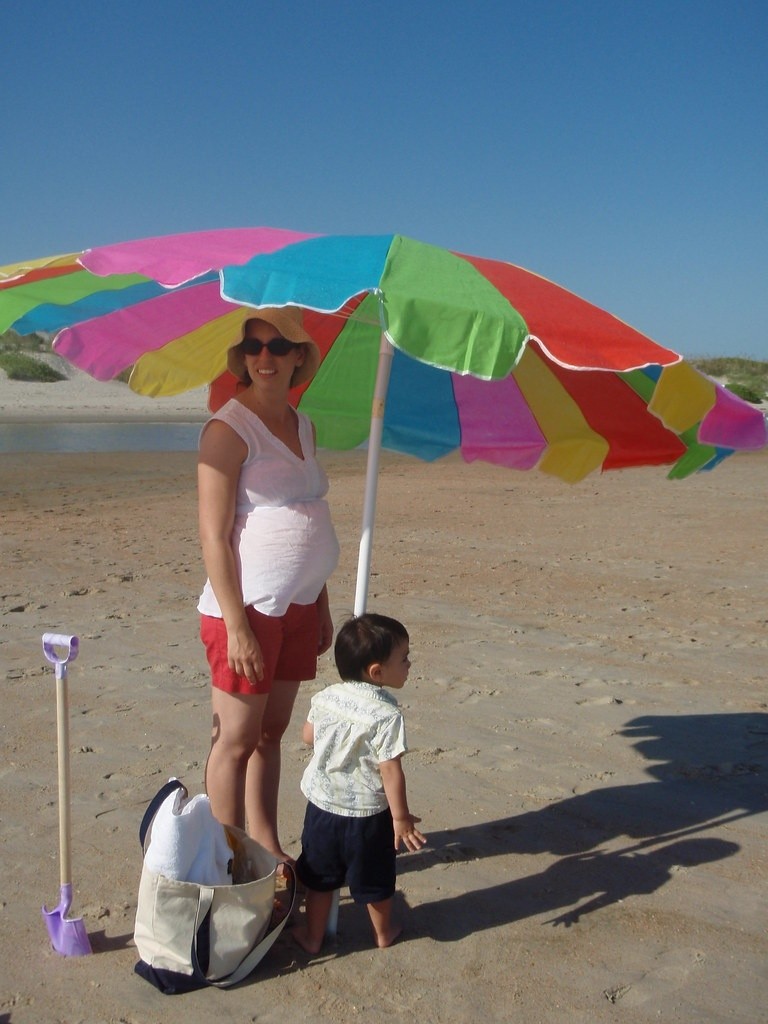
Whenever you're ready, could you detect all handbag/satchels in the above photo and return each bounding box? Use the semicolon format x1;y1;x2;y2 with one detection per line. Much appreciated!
133;779;297;995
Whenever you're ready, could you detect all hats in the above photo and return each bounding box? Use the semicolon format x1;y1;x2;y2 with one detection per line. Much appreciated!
228;304;321;389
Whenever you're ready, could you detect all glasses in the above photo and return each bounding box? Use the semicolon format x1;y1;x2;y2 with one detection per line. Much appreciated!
240;337;299;357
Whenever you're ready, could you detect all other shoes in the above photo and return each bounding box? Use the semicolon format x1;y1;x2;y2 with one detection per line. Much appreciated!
274;860;306;894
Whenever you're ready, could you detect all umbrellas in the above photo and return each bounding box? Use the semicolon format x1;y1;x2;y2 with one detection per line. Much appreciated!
0;227;768;935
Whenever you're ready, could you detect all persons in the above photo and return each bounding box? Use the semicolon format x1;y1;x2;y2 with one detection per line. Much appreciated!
288;611;429;955
194;302;343;926
